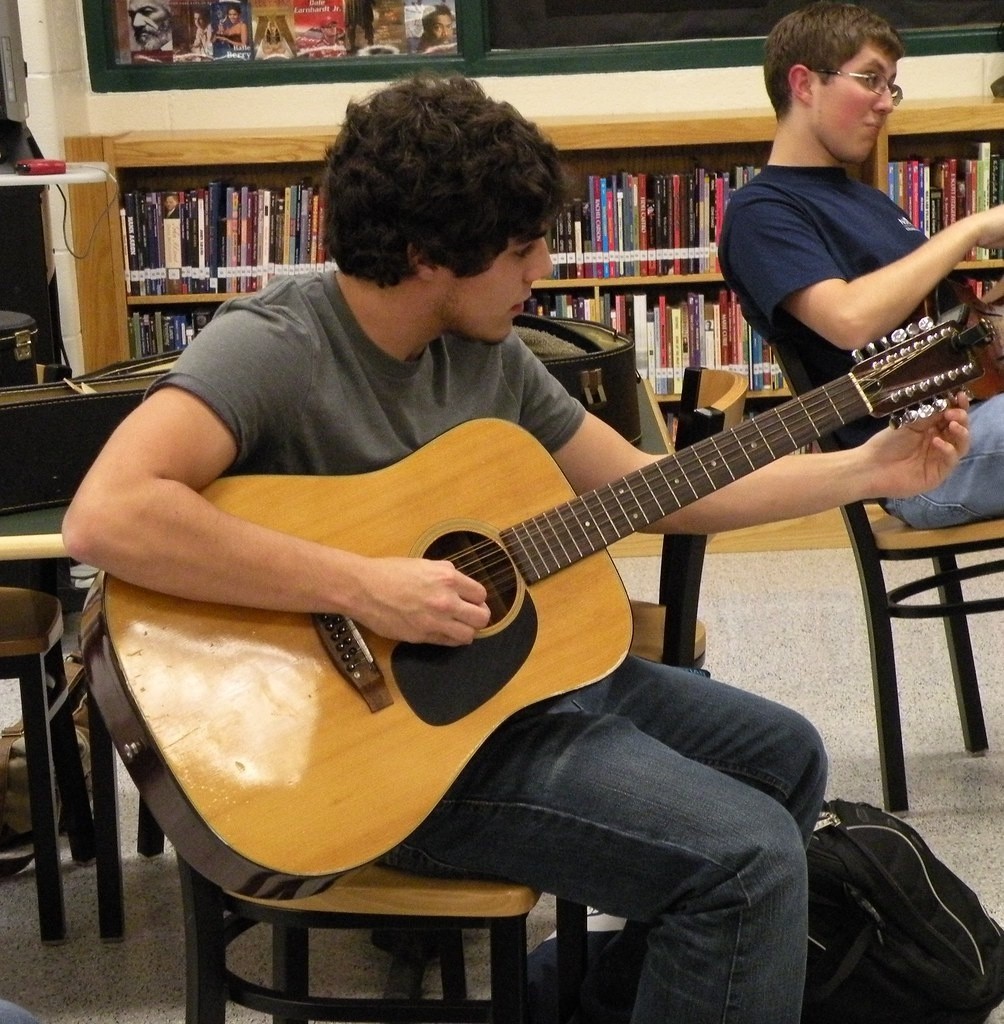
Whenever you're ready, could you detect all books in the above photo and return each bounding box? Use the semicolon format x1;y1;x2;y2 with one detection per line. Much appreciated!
529;286;789;396
120;182;335;296
545;162;762;280
965;276;998;299
114;0;460;66
126;310;213;361
888;142;1004;262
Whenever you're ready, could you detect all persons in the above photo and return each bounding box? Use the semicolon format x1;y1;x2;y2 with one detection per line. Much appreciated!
163;194;180;219
190;8;214;55
60;74;971;1024
716;1;1004;528
415;4;455;52
214;3;248;47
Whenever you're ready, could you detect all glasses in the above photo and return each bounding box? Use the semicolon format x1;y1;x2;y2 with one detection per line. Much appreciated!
808;66;903;106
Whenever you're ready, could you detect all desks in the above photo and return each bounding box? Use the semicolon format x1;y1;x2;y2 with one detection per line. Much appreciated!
0;530;129;943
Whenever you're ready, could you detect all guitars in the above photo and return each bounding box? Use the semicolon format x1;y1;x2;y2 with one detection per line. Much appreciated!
902;268;1004;406
76;302;998;902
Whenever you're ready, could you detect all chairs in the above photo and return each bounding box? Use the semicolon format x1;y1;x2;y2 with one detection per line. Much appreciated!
773;347;1003;812
555;363;748;936
177;855;542;1024
0;587;92;945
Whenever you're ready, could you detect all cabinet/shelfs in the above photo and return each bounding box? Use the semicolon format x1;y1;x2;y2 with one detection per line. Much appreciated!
61;98;1004;560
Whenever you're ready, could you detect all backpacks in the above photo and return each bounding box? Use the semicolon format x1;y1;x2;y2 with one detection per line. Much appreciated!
802;796;1004;1024
